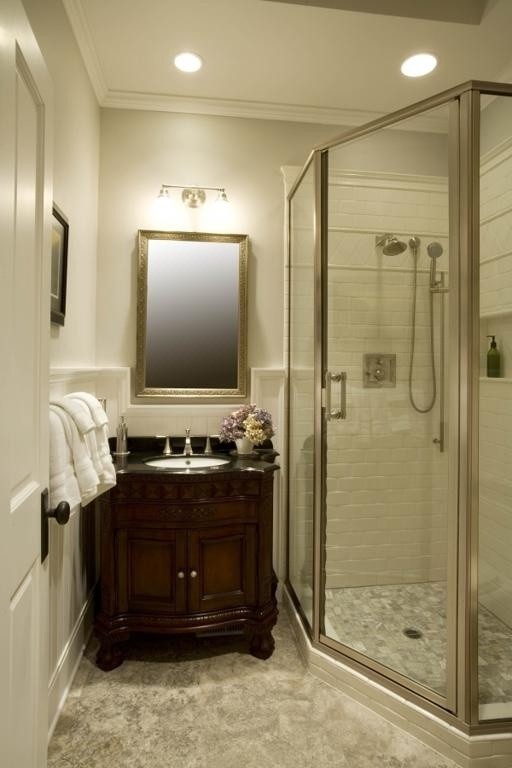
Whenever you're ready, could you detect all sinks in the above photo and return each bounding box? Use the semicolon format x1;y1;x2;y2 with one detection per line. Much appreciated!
143;455;232;468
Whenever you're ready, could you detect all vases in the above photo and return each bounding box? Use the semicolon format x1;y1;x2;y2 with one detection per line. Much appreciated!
235;437;254;454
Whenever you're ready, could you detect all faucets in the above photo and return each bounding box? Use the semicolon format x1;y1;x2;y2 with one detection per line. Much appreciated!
183;429;193;455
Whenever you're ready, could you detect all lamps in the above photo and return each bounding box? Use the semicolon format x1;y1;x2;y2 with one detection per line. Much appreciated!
149;184;234;232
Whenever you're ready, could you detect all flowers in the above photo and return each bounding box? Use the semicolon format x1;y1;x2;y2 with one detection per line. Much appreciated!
219;404;275;447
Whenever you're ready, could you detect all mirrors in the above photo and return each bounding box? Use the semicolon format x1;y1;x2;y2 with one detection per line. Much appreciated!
135;230;249;397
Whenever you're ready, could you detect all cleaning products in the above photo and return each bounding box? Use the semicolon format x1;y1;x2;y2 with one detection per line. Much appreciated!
115;415;128;454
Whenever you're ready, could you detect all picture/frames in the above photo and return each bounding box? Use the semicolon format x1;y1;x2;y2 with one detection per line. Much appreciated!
51;201;69;327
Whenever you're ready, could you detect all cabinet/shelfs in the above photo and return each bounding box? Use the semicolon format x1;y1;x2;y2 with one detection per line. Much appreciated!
93;470;279;672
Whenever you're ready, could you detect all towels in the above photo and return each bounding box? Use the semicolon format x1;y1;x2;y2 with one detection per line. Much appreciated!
50;391;117;517
